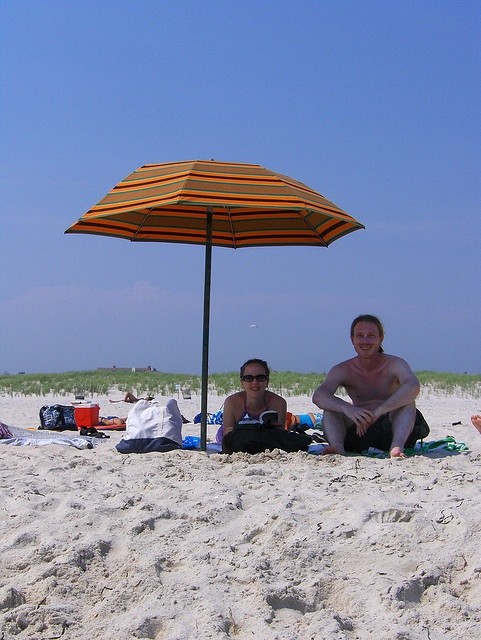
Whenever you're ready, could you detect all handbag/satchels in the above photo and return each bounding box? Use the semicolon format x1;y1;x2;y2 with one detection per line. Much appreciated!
115;399;182;454
40;404;78;431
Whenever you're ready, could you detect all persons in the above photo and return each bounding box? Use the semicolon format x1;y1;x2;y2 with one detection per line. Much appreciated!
64;157;366;452
108;392;155;403
313;314;421;459
471;413;481;434
223;358;288;438
99;416;129;425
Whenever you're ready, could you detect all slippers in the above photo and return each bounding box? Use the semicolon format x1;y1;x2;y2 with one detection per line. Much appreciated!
81;428;110;439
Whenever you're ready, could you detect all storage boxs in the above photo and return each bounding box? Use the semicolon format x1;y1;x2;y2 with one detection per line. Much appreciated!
73;404;100;427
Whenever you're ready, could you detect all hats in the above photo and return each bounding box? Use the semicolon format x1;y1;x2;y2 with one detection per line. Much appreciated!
241;375;268;381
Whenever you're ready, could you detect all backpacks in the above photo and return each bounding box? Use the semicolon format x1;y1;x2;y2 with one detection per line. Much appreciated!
222;422;313;455
